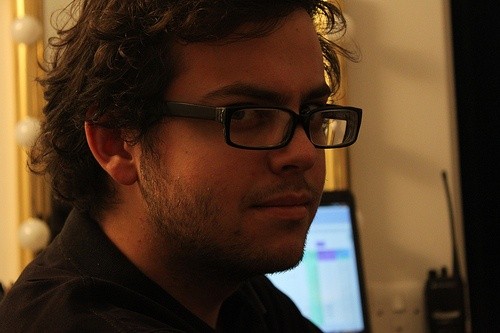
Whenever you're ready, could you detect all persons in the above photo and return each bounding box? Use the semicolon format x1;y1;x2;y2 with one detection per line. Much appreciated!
0;0;362;333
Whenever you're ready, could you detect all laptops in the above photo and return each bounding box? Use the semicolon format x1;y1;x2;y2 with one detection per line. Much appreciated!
264;190;372;333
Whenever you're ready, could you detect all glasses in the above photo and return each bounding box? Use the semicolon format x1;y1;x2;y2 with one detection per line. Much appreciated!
162;101;364;150
